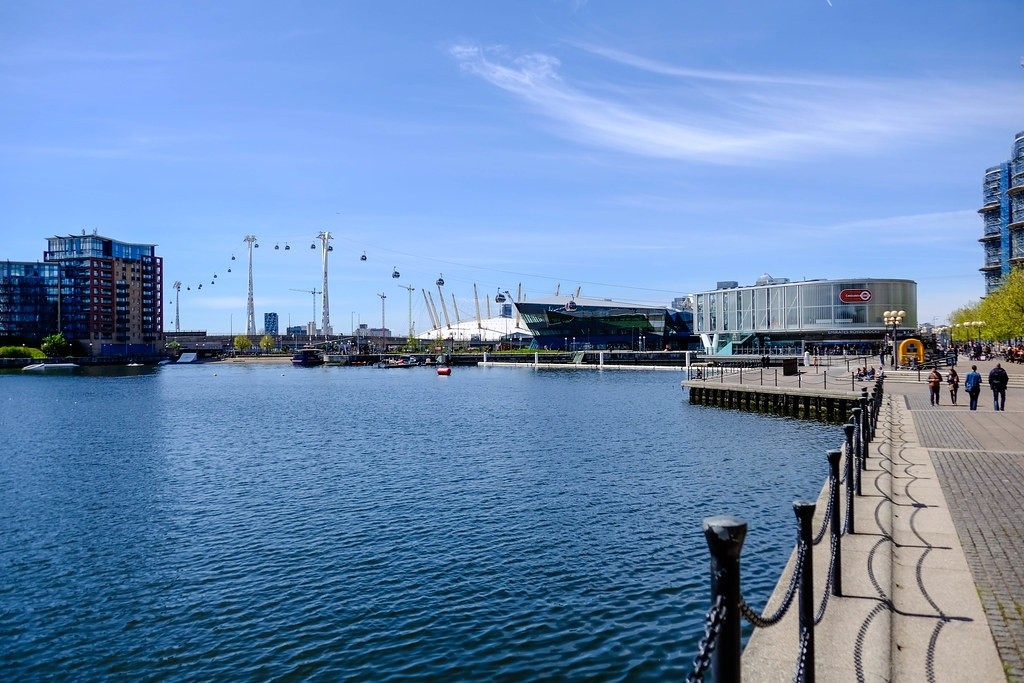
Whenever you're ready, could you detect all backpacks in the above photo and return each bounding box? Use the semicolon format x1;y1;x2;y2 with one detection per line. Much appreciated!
991;368;1004;386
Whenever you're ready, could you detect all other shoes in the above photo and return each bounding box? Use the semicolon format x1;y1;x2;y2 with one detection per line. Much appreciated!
1001;407;1004;411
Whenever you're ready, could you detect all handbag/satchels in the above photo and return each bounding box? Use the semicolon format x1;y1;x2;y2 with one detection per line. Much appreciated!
948;384;954;391
965;383;972;392
970;386;980;399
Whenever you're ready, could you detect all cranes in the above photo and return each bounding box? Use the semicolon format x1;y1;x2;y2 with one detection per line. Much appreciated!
398;284;415;338
287;288;321;323
377;291;387;347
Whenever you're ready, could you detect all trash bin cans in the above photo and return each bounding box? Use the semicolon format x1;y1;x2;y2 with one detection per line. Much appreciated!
946;352;956;365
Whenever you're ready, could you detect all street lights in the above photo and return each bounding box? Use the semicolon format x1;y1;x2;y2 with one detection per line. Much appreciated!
883;310;906;370
933;323;961;348
964;322;972;341
972;321;986;344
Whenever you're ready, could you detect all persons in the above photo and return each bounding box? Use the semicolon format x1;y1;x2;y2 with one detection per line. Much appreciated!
989;363;1009;411
772;344;794;356
960;344;967;349
855;366;875;380
879;349;883;365
970;342;991;360
1001;344;1024;364
878;365;884;376
762;355;770;369
927;367;942;406
804;344;856;355
947;369;959;404
965;365;982;410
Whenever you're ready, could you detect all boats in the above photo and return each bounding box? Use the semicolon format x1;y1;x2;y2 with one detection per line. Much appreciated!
125;363;145;367
377;354;416;365
290;345;330;367
22;362;81;372
